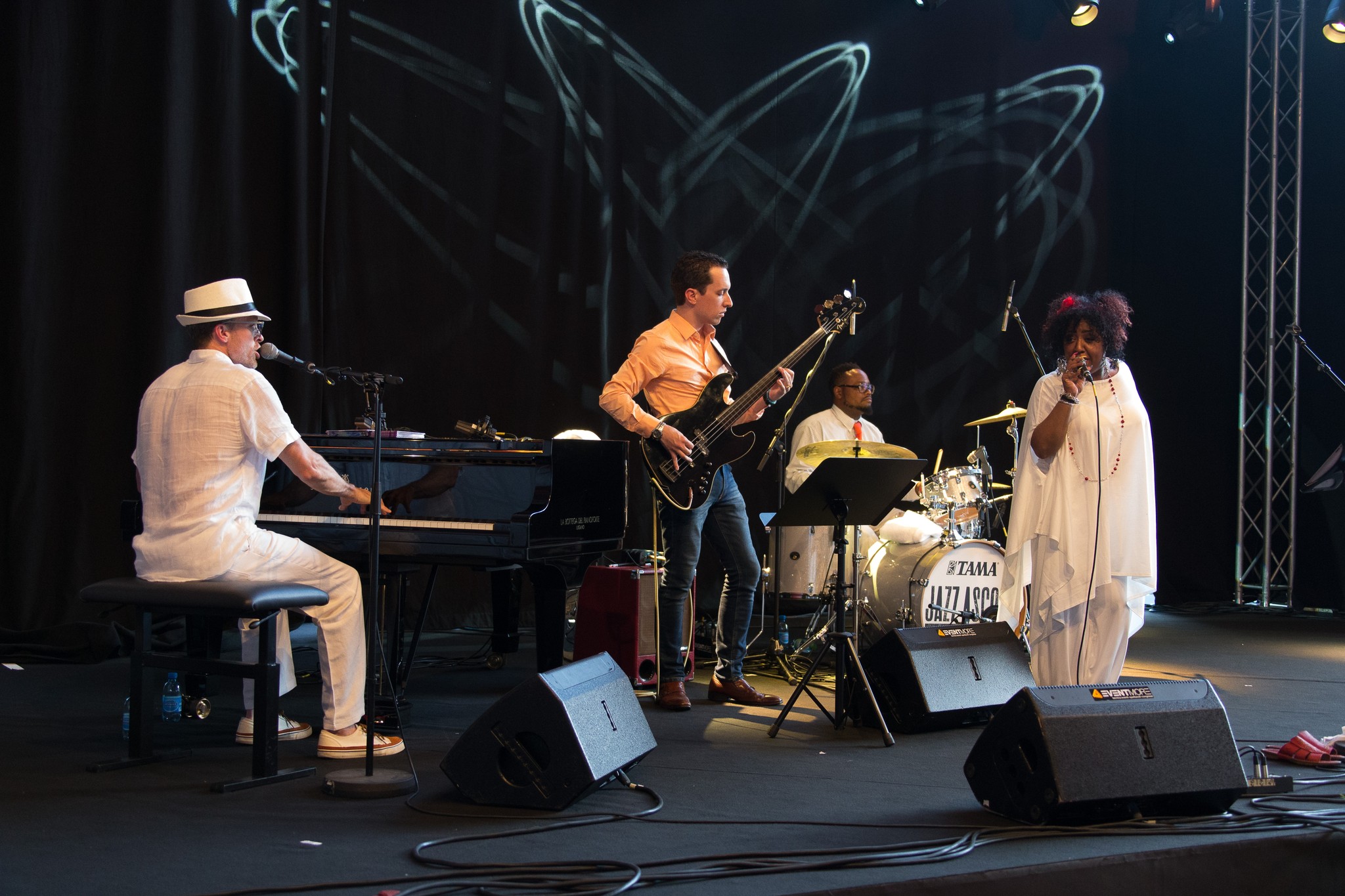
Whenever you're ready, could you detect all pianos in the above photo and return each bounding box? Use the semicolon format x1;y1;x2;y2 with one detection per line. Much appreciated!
244;428;631;682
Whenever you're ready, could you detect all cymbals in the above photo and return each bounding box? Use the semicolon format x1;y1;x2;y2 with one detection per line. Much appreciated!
977;481;1013;490
795;437;920;468
961;398;1028;427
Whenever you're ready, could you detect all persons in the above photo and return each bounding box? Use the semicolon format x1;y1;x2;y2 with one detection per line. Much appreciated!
130;277;403;759
597;250;782;711
997;290;1157;687
782;361;923;539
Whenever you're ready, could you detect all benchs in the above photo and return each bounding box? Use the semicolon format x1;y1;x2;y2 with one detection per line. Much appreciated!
76;580;329;794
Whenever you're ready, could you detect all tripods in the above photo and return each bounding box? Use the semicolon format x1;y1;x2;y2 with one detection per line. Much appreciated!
767;457;930;747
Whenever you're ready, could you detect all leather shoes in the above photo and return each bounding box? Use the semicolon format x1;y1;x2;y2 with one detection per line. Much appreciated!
708;673;783;705
659;681;691;709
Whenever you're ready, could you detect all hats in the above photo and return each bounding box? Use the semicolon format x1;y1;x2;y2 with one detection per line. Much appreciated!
176;278;271;327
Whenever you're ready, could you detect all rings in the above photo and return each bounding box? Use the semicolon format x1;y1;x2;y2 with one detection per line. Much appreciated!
784;383;791;389
1064;369;1071;374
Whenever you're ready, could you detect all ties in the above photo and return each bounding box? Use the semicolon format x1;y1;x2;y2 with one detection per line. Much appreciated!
854;422;862;441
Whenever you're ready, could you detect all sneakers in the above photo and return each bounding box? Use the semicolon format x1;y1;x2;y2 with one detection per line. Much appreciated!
236;712;312;745
318;724;405;759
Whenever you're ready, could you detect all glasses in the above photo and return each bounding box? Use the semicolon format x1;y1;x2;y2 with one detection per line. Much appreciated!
221;321;265;333
839;383;874;394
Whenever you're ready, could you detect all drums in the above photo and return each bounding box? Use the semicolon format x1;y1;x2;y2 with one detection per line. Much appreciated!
914;463;993;528
857;534;1029;643
762;517;862;601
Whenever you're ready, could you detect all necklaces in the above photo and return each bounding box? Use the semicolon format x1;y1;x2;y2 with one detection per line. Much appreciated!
1065;359;1125;482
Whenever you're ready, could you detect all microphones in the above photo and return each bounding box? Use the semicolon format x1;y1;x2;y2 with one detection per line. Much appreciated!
261;342;328;385
1072;357;1093;383
967;445;986;463
1000;280;1015;331
455;420;508;442
849;279;857;335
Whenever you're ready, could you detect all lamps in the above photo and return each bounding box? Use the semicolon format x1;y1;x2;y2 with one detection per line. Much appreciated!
1056;0;1099;27
1164;22;1189;45
1322;0;1345;43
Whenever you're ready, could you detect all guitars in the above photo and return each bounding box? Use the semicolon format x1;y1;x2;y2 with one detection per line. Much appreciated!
639;277;867;511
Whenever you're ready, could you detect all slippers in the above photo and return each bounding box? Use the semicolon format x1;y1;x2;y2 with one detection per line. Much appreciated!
1266;731;1345;759
1260;736;1342;766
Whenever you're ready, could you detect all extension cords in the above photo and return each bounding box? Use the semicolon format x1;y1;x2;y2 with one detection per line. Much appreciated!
1236;774;1296;798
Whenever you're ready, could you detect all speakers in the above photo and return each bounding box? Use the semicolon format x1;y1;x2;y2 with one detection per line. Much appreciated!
962;680;1247;827
439;650;657;813
573;563;696;699
844;622;1038;736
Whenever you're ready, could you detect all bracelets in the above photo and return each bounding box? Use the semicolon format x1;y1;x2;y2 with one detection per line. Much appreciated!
1058;391;1080;406
763;389;777;407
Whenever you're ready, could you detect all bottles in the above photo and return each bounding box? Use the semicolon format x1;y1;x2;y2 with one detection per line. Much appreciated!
121;690;130;740
163;671;182;726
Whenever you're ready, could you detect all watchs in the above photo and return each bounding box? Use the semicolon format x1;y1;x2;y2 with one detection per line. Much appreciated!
651;420;666;441
